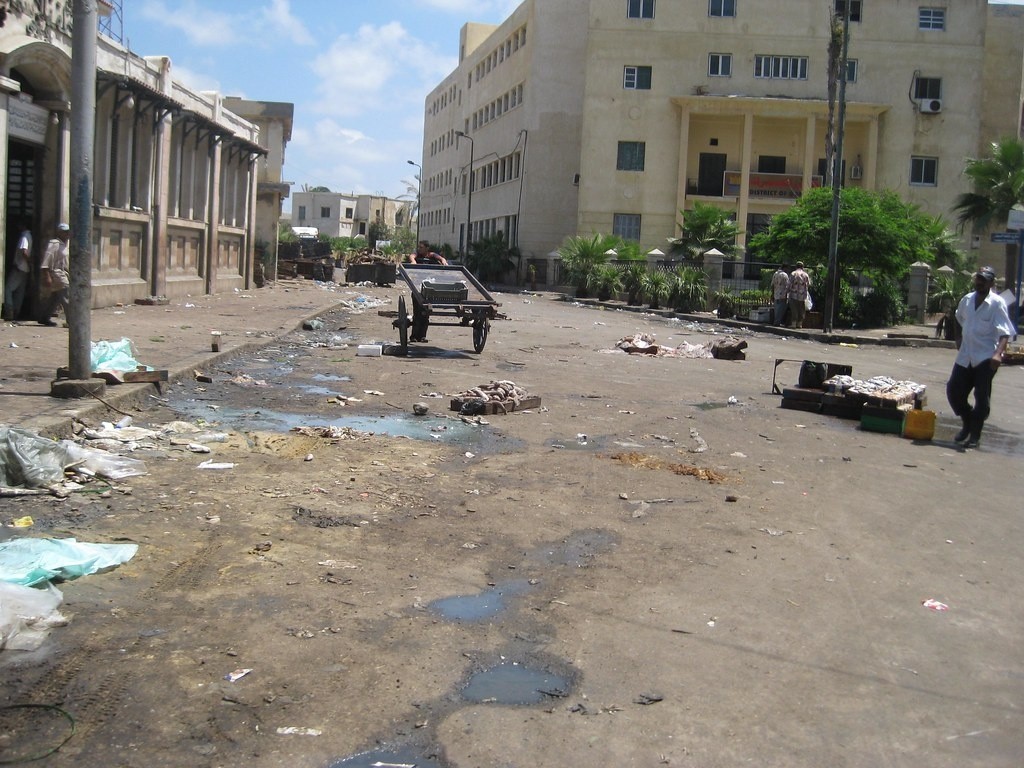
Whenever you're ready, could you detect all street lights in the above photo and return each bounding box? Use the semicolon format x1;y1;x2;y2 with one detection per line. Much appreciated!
408;160;421;251
454;130;473;266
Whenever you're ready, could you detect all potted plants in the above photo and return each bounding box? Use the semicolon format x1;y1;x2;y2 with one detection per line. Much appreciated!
590;266;626;302
710;283;738;319
554;232;623;298
665;264;708;314
638;269;671;310
617;260;650;306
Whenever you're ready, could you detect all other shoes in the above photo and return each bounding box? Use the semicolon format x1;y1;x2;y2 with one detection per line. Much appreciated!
787;325;802;329
410;337;429;343
39;319;57;327
954;419;973;442
963;435;980;448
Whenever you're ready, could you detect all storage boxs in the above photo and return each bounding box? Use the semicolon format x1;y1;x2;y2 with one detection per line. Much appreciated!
781;359;936;440
420;280;469;302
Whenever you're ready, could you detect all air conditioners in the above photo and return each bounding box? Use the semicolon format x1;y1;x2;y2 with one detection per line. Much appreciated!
920;98;943;115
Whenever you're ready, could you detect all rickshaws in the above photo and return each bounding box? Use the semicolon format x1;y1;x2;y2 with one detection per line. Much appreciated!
392;263;497;356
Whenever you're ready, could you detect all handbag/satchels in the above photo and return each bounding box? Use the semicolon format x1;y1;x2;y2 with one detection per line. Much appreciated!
805;290;812;311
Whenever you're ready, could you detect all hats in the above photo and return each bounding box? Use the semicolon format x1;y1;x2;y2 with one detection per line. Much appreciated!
795;261;804;267
57;222;69;231
975;266;995;282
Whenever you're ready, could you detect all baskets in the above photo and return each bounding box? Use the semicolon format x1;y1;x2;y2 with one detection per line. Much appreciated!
421;280;468;301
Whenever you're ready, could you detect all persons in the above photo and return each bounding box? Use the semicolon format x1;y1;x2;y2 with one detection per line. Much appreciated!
2;215;32;320
771;262;789;327
945;265;1016;448
409;240;449;342
788;260;810;329
38;223;70;326
